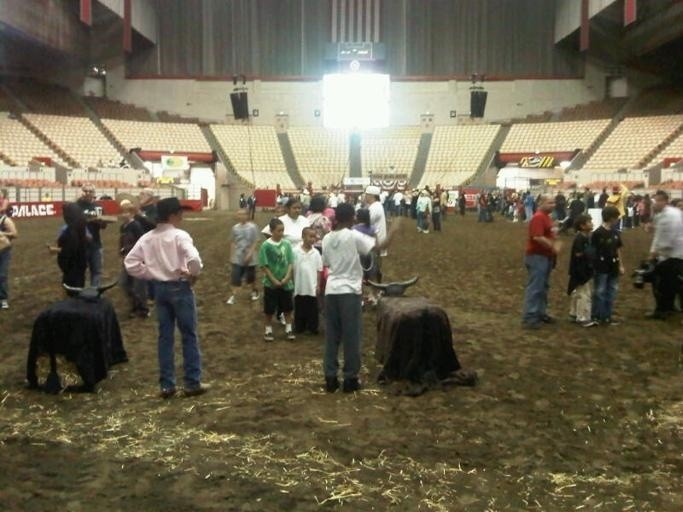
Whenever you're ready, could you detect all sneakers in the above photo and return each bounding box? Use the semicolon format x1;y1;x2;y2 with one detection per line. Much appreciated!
540;312;560;323
250;290;261;301
326;374;361;392
226;294;238;306
571;312;624;329
160;382;212;396
284;329;296;341
262;331;276;343
1;299;8;309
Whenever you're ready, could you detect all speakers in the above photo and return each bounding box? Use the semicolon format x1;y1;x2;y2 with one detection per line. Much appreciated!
471;91;487;117
231;92;249;119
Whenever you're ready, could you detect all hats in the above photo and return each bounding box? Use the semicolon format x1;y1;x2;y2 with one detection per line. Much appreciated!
612;185;619;193
366;186;381;196
151;196;193;218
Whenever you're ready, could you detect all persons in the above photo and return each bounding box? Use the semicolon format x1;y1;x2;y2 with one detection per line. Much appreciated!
477;185;656;230
352;184;388;309
0;200;18;310
241;183;466;234
642;189;683;319
319;204;403;392
54;182;159;319
520;193;624;330
123;198;211;396
225;208;323;343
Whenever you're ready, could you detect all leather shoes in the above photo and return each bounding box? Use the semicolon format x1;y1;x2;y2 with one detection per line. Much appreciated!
644;310;667;321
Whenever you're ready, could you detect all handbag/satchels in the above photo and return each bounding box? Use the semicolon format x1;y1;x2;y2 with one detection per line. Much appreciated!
0;235;12;253
